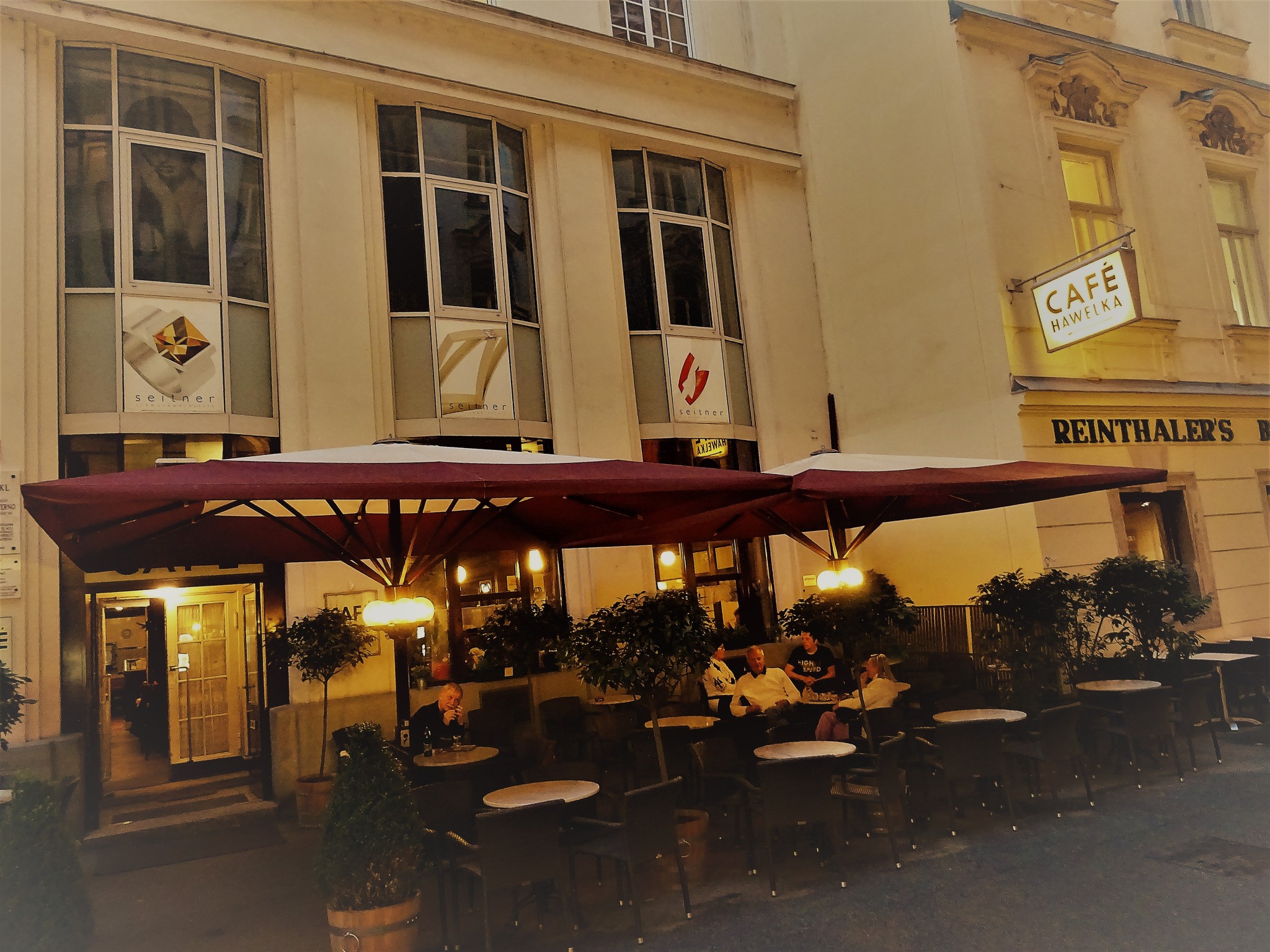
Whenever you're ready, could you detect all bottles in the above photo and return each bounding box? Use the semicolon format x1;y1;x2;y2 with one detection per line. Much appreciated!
423;723;433;757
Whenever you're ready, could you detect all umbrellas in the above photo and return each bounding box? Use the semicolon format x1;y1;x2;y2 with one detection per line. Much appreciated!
20;438;793;727
563;445;1167;571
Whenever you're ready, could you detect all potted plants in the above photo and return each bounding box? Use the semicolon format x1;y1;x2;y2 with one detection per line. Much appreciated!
315;721;429;952
257;605;378;830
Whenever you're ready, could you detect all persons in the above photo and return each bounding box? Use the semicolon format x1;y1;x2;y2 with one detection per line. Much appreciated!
701;639;738;712
94;96;244;285
730;645;801;716
785;629;836;686
816;654;898;741
410;682;465;748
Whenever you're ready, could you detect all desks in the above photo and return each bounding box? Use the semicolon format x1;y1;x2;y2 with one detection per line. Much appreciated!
1075;680;1162;774
1189;652;1262;731
644;716;720;807
753;742;857;849
932;708;1026;811
586;694;641;739
413;746;499;843
483;780;599;930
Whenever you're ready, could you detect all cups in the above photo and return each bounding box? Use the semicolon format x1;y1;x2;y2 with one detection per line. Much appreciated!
810;692;838;701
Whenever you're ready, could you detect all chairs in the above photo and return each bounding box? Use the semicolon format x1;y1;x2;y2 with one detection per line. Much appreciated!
413;636;1270;952
